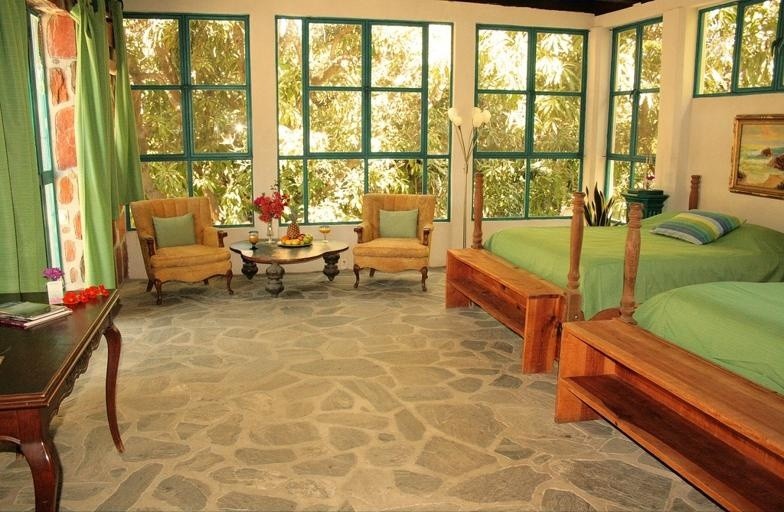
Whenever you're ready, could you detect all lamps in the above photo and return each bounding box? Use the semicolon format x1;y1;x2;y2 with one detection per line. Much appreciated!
445;108;493;250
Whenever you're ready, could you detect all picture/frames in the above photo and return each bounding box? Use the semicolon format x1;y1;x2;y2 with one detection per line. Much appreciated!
728;113;784;200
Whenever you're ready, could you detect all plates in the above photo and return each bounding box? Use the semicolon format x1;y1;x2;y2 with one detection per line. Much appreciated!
276;241;313;248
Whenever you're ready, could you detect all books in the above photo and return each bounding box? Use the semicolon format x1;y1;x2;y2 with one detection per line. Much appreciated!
0;301;73;330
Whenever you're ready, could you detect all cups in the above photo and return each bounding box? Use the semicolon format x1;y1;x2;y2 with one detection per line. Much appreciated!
318;226;330;243
247;230;259;250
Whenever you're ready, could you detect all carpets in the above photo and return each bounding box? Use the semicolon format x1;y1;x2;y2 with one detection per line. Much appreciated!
0;272;784;512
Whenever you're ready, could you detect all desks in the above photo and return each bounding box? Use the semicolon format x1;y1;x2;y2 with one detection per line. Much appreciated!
0;286;126;512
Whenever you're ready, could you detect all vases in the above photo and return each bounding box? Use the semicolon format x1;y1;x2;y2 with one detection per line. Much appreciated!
46;282;64;306
266;222;274;243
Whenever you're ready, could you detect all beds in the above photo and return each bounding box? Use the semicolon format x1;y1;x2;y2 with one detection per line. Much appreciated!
619;203;784;396
474;174;784;362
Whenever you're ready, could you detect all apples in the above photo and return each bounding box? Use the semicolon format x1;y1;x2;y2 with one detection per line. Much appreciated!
303;237;310;244
305;233;313;241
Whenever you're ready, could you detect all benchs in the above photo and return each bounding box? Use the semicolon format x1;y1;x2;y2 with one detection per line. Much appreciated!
445;249;566;372
554;318;784;512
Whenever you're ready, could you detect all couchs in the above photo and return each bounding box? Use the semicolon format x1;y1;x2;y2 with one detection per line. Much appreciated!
353;193;436;292
130;198;233;306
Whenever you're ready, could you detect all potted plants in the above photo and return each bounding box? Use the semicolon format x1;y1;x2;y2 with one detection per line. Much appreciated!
580;182;621;226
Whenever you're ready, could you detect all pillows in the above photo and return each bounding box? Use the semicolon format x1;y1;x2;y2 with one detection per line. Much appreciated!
379;208;418;238
152;213;196;249
649;209;747;245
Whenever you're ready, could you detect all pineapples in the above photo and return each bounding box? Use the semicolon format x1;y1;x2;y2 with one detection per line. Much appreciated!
287;213;300;240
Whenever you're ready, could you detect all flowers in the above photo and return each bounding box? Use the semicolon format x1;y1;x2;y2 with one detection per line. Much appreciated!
41;267;65;281
247;177;290;223
62;284;109;304
